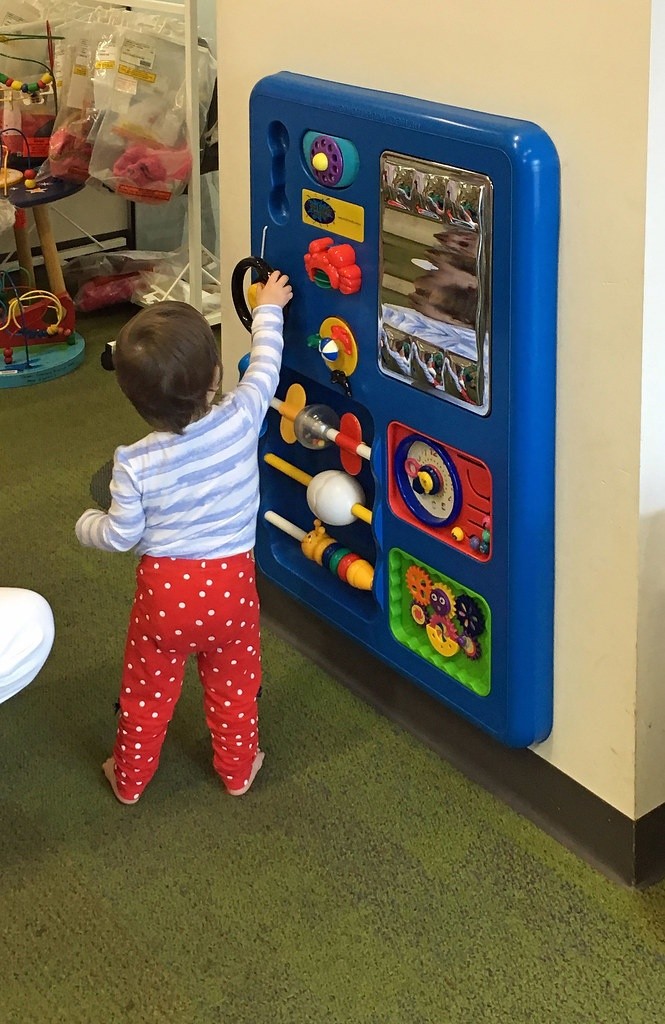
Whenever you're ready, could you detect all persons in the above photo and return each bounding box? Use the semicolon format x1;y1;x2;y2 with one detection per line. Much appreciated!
72;269;296;808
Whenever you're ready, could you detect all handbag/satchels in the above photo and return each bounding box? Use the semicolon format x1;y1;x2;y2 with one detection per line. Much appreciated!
0;0;216;207
63;244;223;329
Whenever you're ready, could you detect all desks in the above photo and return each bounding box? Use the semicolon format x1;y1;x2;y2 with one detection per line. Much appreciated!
6;164;86;294
